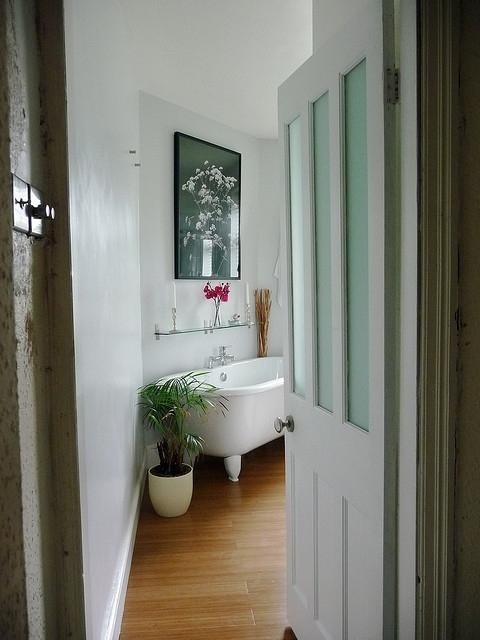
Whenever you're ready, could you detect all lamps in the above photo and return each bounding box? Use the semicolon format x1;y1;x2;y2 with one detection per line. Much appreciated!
31;202;57;221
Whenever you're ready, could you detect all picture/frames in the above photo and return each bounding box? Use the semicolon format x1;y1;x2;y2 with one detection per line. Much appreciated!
172;130;242;281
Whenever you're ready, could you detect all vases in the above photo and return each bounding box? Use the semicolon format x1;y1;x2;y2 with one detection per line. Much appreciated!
202;240;213;278
211;304;222;327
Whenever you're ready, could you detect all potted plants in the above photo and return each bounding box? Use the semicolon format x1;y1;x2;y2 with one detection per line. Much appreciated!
134;367;230;520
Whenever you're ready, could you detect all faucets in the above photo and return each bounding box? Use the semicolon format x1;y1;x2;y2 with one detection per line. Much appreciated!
218;346;235;365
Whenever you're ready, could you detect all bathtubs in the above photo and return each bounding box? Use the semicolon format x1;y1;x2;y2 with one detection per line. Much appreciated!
157;356;285;482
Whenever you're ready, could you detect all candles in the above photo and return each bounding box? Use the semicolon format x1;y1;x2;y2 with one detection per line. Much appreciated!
172;284;176;308
245;283;250;305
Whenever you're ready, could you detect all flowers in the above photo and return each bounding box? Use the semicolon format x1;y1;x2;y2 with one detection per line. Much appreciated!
181;160;237;253
202;280;231;325
